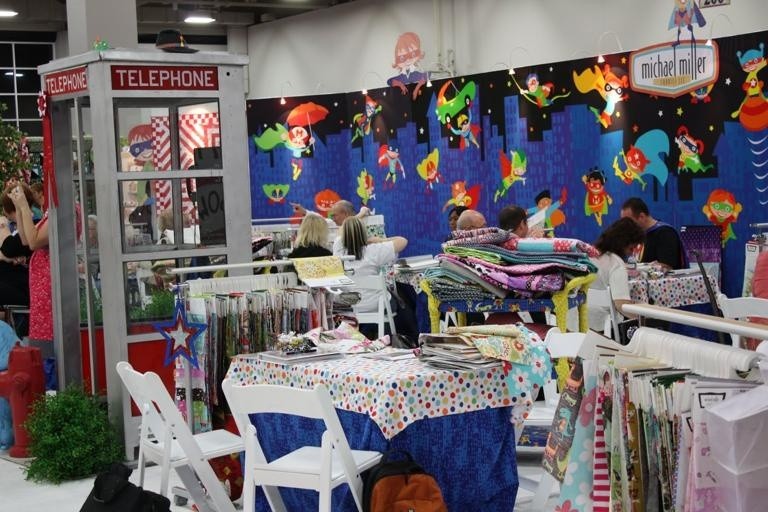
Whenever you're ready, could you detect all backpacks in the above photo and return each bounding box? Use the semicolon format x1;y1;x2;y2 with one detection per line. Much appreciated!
362;449;447;512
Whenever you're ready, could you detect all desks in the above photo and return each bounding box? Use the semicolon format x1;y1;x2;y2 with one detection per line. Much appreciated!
418;272;596;393
391;263;465;337
627;272;720;343
221;344;551;512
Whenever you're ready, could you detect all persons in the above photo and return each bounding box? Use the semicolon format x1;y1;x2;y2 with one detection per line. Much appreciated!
0;175;102;451
286;200;417;337
447;206;543;239
621;198;689;269
563;217;643;329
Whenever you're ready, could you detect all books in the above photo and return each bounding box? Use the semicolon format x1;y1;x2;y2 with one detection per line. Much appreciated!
397;255;440;268
418;331;505;371
256;347;344;365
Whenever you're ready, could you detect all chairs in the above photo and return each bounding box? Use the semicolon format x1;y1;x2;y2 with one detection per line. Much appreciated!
716;292;768;351
560;285;621;344
221;377;384;512
350;272;397;340
115;360;242;512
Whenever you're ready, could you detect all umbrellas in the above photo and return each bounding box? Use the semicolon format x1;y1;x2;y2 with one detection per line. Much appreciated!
285;101;330;150
